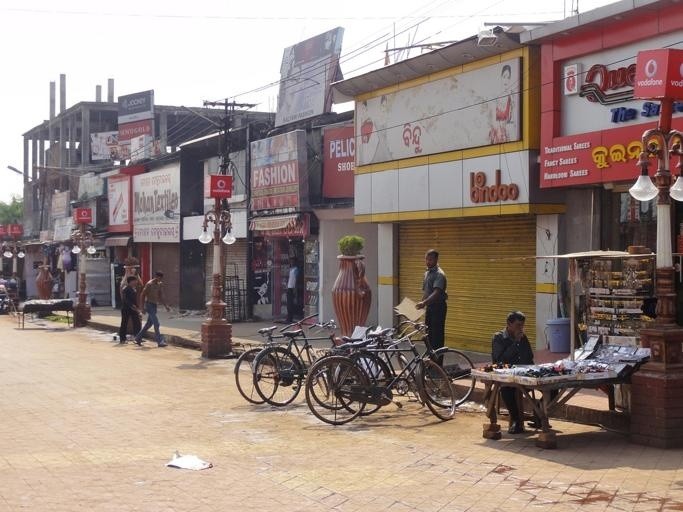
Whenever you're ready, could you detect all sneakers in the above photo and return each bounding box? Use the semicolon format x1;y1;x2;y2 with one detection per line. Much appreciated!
158;341;168;347
135;339;144;346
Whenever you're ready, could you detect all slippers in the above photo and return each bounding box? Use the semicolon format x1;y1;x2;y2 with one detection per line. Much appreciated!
527;417;552;428
508;421;524;433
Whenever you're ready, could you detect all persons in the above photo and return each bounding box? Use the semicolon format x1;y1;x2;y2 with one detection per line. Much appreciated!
488;65;515;144
119;277;145;344
287;258;302;329
415;247;447;380
134;270;171;348
371;95;395;162
358;99;373;162
491;311;560;433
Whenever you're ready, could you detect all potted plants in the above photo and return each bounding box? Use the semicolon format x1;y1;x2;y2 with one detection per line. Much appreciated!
331;235;372;353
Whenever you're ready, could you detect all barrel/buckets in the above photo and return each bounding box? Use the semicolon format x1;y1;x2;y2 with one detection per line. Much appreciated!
546;316;571;354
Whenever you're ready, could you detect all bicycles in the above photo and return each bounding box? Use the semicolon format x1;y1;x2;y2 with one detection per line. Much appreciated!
226;320;474;424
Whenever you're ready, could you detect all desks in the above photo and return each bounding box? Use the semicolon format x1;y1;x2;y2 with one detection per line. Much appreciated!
470;366;632;431
21;299;75;328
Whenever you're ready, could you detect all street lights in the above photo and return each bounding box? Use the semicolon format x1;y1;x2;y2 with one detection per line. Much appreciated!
1;250;27;316
71;231;97;329
198;209;237;360
628;128;682;452
